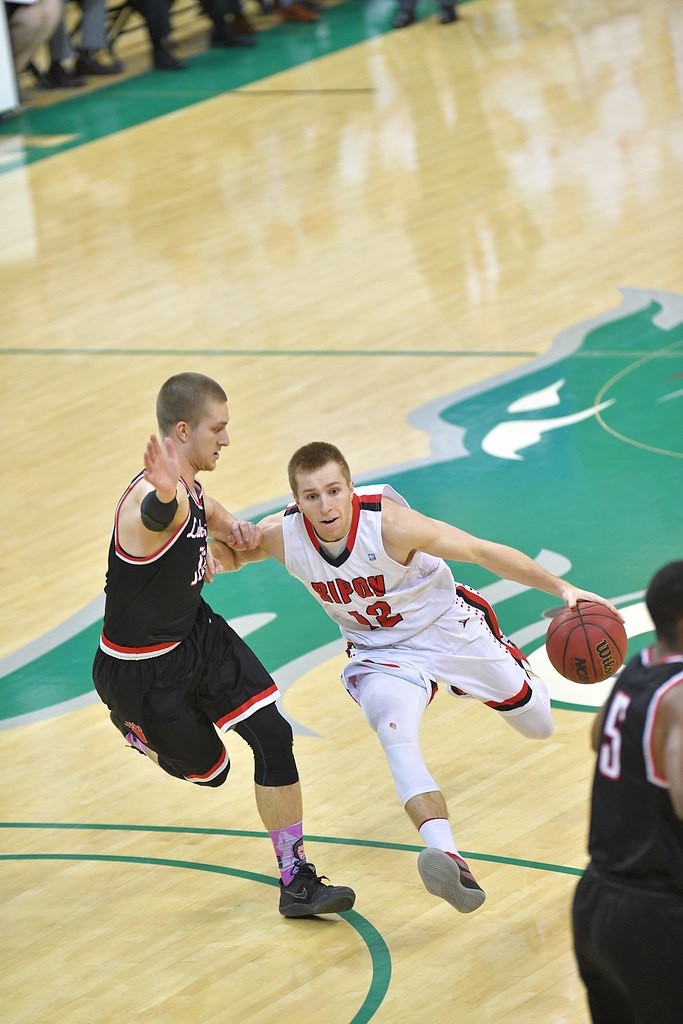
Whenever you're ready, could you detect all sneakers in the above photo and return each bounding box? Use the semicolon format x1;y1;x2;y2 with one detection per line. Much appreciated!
417;847;485;914
279;860;356;917
109;710;147;757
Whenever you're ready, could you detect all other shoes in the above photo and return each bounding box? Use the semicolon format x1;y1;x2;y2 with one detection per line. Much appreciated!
14;0;478;104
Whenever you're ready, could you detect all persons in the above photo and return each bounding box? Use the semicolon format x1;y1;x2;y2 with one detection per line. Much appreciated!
93;371;356;918
572;559;682;1024
389;0;458;31
5;1;322;88
202;443;625;913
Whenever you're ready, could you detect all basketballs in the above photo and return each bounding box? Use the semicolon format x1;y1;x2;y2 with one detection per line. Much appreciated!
544;597;630;685
